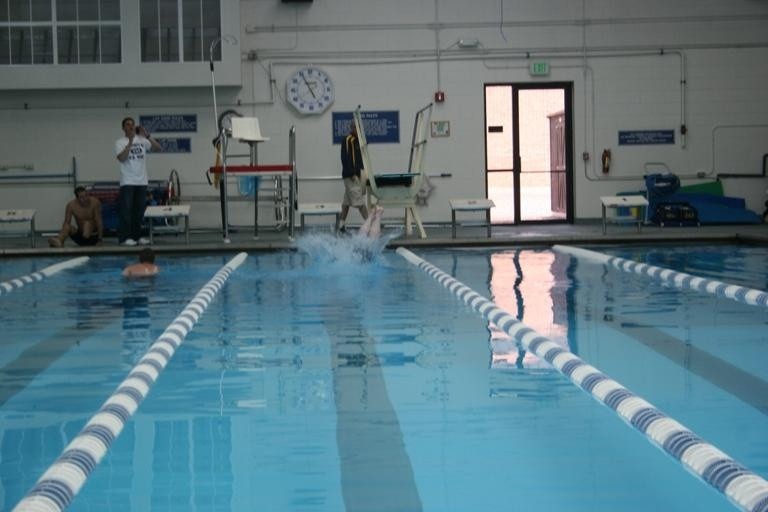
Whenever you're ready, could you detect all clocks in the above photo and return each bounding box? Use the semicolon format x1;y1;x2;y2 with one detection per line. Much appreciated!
285;67;336;118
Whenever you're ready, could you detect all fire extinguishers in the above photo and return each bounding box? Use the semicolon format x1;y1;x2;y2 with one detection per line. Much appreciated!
602;149;611;172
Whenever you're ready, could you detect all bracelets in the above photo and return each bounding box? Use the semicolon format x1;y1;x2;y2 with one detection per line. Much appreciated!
147;134;150;138
98;239;102;242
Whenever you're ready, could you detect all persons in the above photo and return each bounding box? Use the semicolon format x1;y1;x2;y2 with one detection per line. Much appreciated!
120;248;160;280
349;206;385;267
336;119;372;235
48;186;103;247
122;286;151;372
115;117;162;247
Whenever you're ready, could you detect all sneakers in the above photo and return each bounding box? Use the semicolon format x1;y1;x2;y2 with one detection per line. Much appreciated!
138;237;150;245
120;239;137;245
